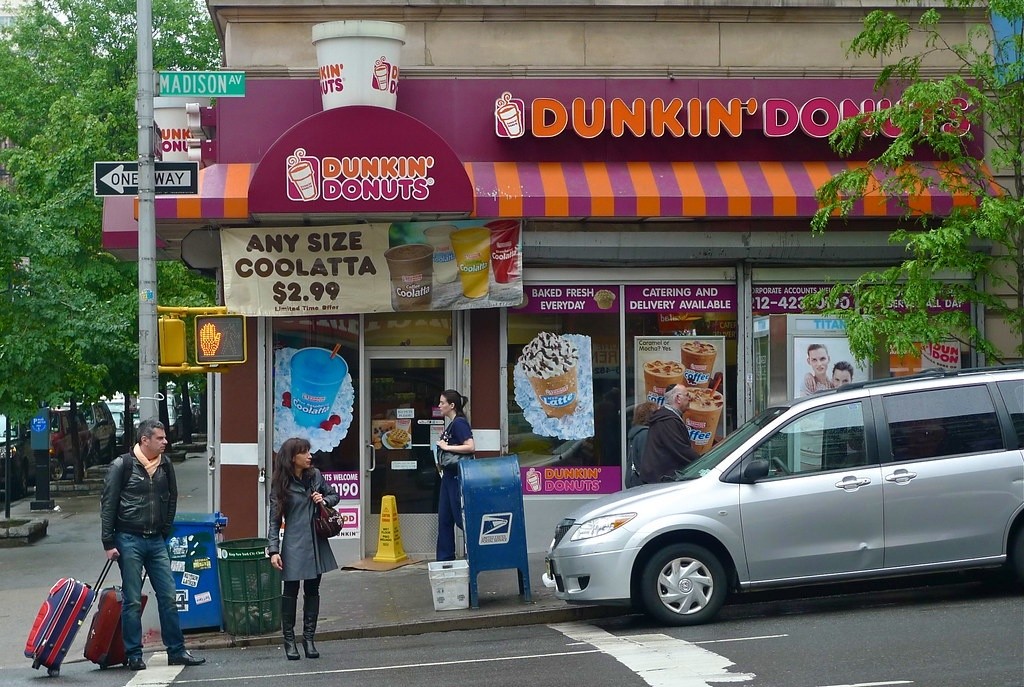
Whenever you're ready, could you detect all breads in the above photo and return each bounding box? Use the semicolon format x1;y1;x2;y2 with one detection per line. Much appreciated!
387;428;410;449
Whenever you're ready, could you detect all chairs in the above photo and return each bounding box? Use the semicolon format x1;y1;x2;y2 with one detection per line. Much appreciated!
841;428;864;467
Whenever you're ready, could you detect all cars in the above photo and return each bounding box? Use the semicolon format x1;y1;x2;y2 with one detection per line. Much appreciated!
0;391;205;502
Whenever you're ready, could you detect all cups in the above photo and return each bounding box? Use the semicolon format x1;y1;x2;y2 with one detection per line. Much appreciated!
289;347;348;428
525;358;579;418
384;220;520;311
642;341;724;455
312;20;406;110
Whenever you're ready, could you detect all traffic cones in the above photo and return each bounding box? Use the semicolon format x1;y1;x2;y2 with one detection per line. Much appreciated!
371;493;410;564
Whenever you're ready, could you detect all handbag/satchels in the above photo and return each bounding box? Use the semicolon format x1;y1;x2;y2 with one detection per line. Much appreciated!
310;464;344;541
438;417;476;470
631;470;646;487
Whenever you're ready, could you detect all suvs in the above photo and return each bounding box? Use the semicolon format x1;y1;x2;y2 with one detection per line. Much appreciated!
542;363;1024;627
513;374;786;487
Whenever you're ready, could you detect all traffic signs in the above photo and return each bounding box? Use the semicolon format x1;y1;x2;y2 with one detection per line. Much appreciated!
93;161;199;197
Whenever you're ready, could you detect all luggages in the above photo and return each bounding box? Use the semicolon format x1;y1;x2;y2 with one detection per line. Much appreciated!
84;569;148;669
24;552;119;676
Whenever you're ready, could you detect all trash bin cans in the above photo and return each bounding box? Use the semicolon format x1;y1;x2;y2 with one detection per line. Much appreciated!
427;560;469;611
218;537;284;636
455;453;531;608
164;511;228;636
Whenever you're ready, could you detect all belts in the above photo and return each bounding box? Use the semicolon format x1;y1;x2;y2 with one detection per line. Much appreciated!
119;530;152;539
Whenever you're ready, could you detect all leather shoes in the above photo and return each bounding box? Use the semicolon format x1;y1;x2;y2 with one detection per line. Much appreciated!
168;653;205;665
128;657;145;670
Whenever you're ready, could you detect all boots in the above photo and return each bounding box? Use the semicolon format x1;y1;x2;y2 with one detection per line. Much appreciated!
302;595;320;659
281;594;300;660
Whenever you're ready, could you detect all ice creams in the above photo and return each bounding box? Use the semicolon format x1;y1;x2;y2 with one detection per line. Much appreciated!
519;331;579;419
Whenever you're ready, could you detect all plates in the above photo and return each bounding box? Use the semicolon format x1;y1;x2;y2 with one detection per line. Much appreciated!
382;430;411;449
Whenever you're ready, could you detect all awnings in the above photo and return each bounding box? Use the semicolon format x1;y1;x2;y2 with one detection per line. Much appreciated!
133;163;1003;222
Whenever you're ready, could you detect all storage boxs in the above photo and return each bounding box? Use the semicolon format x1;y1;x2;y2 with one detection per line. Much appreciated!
428;560;470;611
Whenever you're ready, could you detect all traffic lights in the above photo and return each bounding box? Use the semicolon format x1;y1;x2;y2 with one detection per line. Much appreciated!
157;317;189;366
196;312;248;367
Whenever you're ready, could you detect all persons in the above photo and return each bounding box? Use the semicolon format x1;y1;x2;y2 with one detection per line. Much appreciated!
266;438;340;660
436;389;475;562
800;344;854;471
624;401;658;490
638;384;699;486
99;420;207;671
